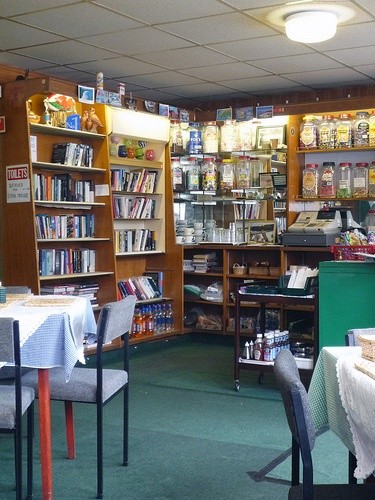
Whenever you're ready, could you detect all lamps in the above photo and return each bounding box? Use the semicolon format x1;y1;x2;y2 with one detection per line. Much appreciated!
266;2;355;44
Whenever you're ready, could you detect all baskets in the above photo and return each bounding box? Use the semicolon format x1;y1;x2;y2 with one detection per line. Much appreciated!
329;243;375;260
355;334;375;362
233;262;247;274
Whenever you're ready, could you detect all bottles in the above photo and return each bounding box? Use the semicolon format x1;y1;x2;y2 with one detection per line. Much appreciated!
169;117;263;197
352;161;375;198
0;282;6;305
243;327;291;362
127;301;174;339
302;164;318;199
299;109;375;149
337;162;352;198
319;162;337;199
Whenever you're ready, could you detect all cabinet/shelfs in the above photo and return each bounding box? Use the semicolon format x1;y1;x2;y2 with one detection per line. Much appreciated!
1;77;375;393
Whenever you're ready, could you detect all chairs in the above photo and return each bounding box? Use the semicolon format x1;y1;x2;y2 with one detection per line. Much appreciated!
270;347;375;500
345;327;375;484
0;286;137;500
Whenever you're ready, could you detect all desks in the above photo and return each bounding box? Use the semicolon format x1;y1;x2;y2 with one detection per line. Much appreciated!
310;348;375;475
0;294;97;500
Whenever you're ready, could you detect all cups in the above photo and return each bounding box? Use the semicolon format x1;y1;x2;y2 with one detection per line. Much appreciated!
109;136;155;161
270;139;279;149
175;218;249;245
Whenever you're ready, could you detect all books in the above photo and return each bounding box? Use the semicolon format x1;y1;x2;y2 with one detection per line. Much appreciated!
32;173;96;203
35;213;95;240
234;193;285;233
114;229;156;252
51;143;92;168
118;271;163;301
39;283;99;308
110;168;158;219
37;248;95;276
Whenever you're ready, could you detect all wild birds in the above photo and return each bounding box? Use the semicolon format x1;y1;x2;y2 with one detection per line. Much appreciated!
81;107;104;133
